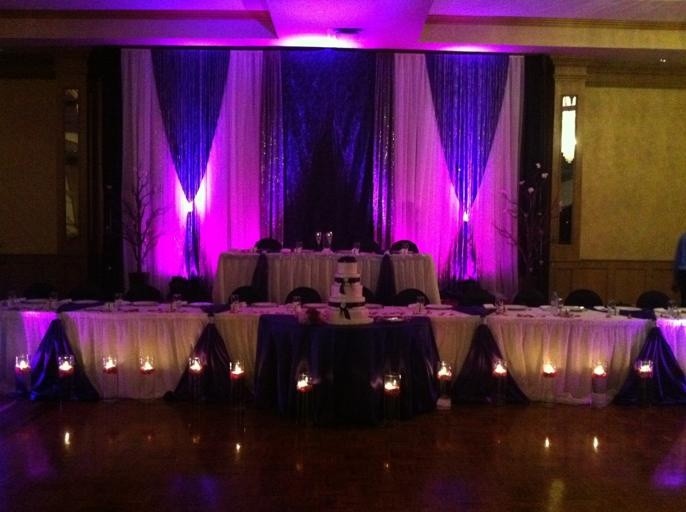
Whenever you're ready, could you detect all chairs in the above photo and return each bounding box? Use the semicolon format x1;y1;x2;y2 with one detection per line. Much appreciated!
254;237;282;253
390;240;419;253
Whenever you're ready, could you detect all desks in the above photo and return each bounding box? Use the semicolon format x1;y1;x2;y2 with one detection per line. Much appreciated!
1;296;686;409
212;244;444;304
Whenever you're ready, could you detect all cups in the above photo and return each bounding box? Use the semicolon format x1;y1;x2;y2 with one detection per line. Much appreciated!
173;293;182;311
231;295;239;305
326;231;333;246
315;231;322;245
293;296;302;311
667;300;678;317
495;295;504;314
416;296;426;313
607;295;616;316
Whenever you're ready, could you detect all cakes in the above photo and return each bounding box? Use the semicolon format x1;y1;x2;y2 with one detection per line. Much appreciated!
327;254;369;325
